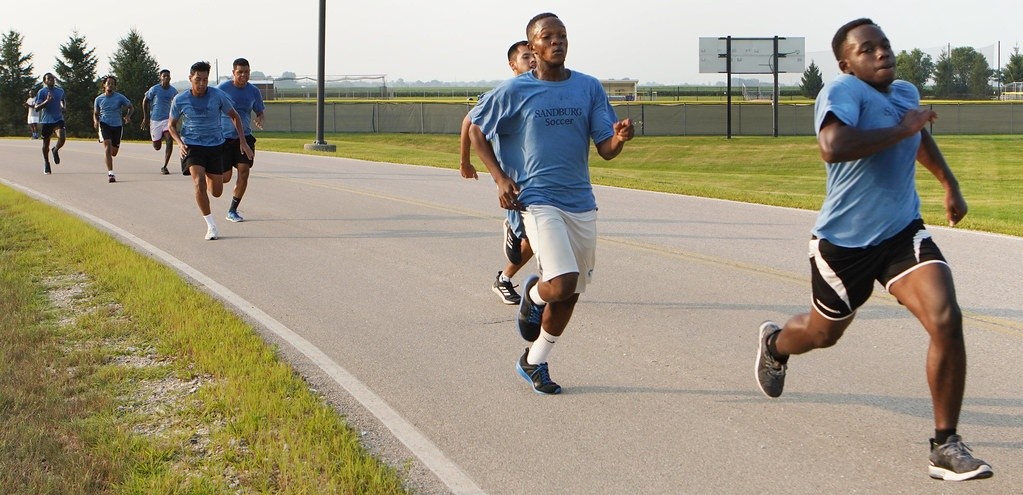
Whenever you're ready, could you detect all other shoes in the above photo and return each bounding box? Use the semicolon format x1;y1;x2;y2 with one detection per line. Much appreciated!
52;147;60;164
32;135;38;139
205;225;219;240
109;174;115;182
45;163;52;175
161;167;169;175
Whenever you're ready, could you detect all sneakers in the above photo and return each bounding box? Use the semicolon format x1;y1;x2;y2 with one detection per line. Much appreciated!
506;226;523;264
929;437;994;481
494;271;521;305
225;212;244;222
519;348;562;397
756;320;788;399
517;276;546;342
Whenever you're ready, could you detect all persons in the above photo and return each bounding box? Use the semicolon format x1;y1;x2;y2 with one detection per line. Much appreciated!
460;13;632;394
168;58;265;240
140;69;178;174
93;77;134;183
34;73;66;175
23;90;39;138
755;18;993;482
